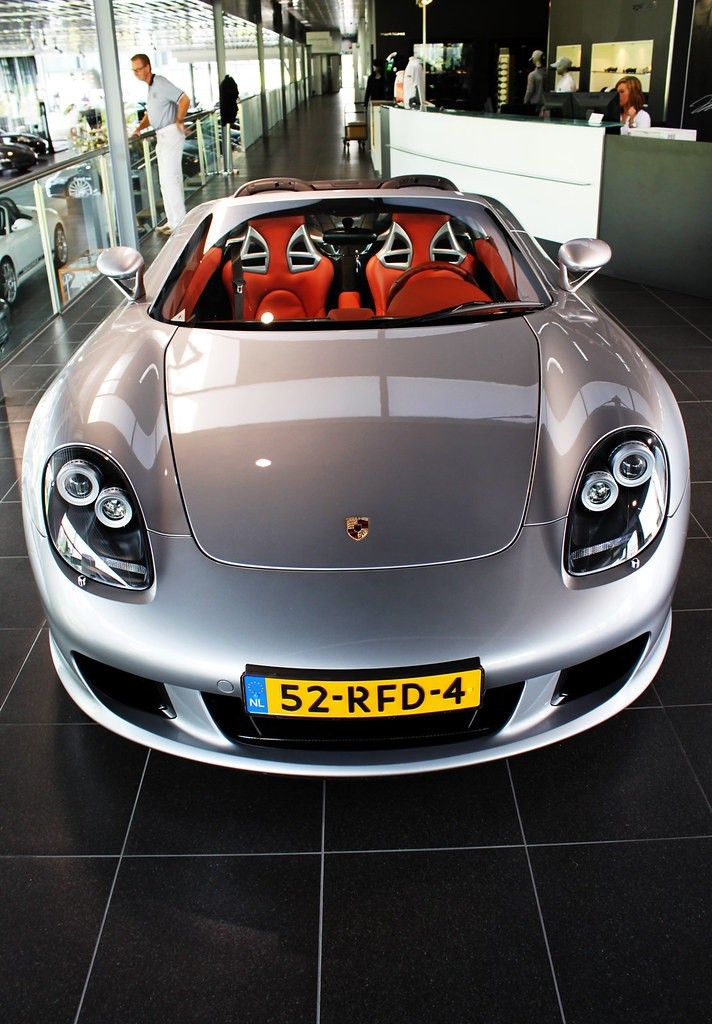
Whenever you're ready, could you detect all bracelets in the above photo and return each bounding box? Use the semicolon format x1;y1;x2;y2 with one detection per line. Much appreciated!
177;119;184;123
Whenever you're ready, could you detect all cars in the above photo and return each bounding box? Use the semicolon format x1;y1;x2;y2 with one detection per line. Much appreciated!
0;143;39;174
44;114;242;198
0;128;49;155
0;196;69;305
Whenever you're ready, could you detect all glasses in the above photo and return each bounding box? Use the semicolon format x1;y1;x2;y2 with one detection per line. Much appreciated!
134;66;147;72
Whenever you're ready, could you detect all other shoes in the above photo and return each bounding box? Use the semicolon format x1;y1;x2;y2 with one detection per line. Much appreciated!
156;221;170;235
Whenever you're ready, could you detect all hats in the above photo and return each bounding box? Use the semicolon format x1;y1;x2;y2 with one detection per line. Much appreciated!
528;50;543;60
550;56;572;67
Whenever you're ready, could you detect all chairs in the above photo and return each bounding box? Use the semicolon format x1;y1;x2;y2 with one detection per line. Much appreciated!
364;175;520;315
176;177;337;324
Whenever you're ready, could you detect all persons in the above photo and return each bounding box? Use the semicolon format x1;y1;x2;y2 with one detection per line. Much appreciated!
404;56;427;111
616;76;651;129
524;51;554;116
551;58;576;93
131;54;191;234
364;59;396;110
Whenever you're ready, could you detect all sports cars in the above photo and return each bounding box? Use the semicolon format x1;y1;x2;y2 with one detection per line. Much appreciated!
20;175;692;778
